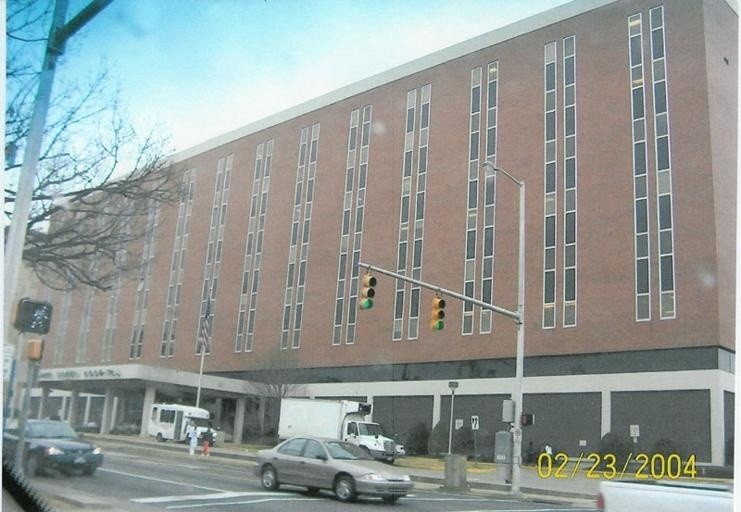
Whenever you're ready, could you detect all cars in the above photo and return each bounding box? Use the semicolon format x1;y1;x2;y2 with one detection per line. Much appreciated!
75;422;139;437
252;436;413;505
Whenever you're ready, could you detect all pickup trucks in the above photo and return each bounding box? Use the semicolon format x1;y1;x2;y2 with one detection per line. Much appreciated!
3;419;103;479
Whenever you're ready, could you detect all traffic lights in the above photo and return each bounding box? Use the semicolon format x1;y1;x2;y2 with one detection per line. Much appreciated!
522;414;533;426
430;297;446;331
358;275;377;310
14;297;52;334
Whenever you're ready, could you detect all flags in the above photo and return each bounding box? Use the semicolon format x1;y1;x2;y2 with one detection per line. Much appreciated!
200;295;212;350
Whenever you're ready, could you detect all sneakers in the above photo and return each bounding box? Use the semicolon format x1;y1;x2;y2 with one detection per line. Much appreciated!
200;453;210;457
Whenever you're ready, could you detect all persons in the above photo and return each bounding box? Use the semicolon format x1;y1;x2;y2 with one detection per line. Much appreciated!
524;440;558;469
202;425;214;457
185;419;202;458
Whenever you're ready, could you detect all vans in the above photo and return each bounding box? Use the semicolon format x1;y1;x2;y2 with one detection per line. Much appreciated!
147;403;217;448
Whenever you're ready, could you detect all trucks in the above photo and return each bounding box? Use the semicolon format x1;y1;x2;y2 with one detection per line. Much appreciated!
277;397;406;465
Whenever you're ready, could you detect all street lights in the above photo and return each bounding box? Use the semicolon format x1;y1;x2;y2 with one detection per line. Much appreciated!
480;161;525;499
